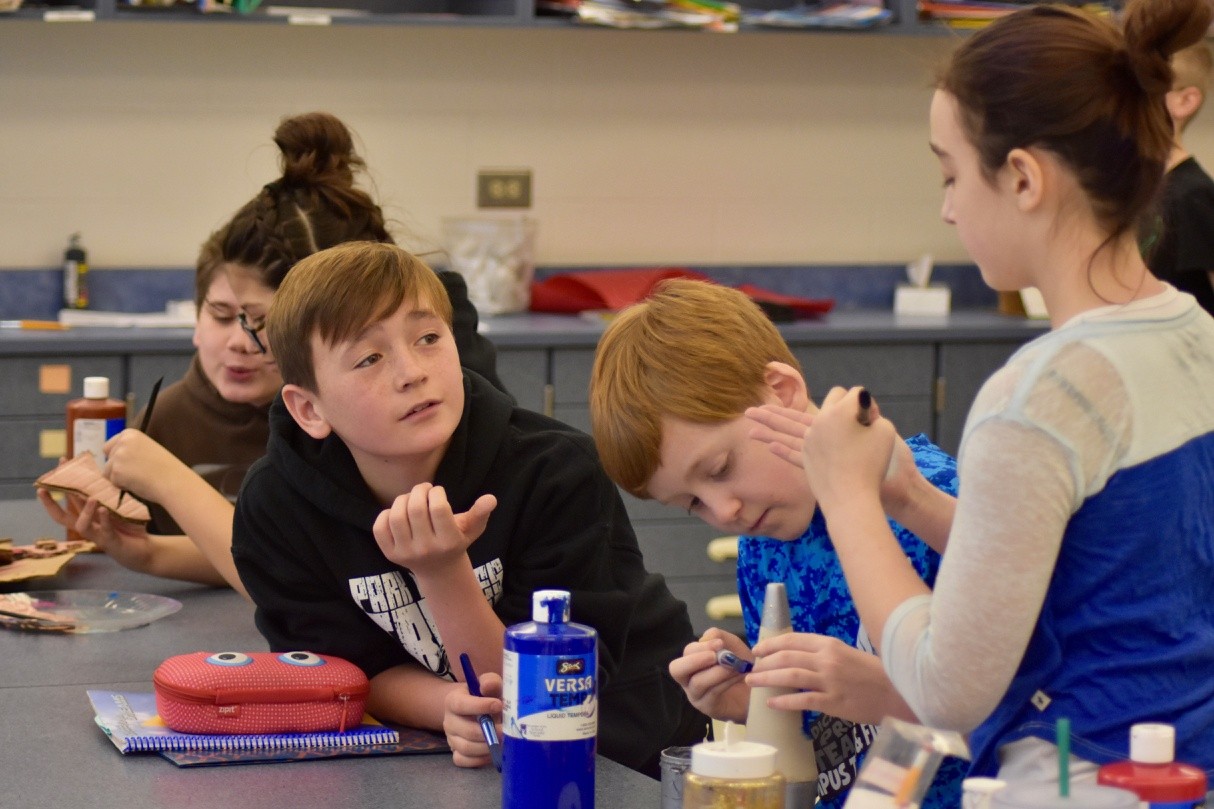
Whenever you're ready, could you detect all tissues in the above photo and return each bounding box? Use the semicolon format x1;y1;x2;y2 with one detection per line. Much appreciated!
891;237;954;317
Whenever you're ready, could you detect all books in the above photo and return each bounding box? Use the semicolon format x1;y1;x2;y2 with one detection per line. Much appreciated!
87;685;398;755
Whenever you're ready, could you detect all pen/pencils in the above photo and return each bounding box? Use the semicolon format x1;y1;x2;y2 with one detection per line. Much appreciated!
459;653;502;772
716;649;754;675
1;320;71;329
892;735;933;809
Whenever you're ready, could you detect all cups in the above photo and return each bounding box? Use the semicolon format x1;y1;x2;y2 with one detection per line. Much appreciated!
991;784;1140;809
659;746;691;809
446;215;536;314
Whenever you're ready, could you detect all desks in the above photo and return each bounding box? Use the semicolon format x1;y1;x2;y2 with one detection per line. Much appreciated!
0;498;667;809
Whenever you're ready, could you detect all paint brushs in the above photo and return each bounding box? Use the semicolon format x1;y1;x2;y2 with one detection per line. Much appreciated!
115;377;163;508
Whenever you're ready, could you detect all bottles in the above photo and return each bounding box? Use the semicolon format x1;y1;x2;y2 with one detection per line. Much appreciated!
63;226;90;309
1098;722;1207;809
501;589;597;809
66;374;128;553
685;720;787;809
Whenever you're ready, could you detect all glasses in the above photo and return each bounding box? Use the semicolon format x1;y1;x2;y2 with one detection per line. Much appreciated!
237;312;267;354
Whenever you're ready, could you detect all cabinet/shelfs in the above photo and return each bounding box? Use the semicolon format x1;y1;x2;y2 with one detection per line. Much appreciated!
0;309;1056;629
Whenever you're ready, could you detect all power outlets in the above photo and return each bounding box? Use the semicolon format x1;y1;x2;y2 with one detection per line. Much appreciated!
478;170;532;211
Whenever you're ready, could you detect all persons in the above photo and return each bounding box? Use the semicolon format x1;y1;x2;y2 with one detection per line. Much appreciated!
587;276;971;809
231;239;717;781
39;113;500;618
746;1;1213;809
